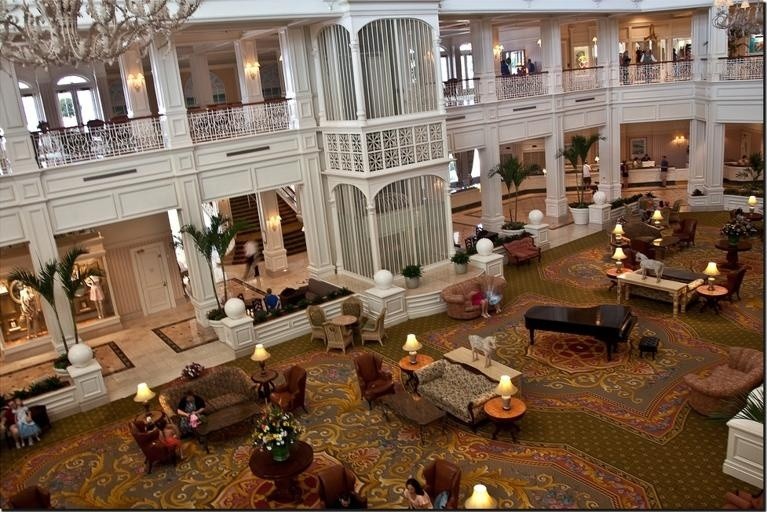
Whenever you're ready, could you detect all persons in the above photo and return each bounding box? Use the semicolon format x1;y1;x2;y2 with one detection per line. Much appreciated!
265;288;280;310
641;154;650;162
19;284;39;339
12;398;41;447
622;50;631;83
637;192;653;221
176;390;208;439
84;274;106;318
659;155;668;188
240;234;259;281
403;476;433;509
737;155;748;165
620;160;629;189
631;157;639;169
502;58;511;75
485;283;503;313
0;400;34;448
655;200;665;211
467;283;491;318
640;48;657;83
156;418;197;462
527;59;534;74
582;160;591;191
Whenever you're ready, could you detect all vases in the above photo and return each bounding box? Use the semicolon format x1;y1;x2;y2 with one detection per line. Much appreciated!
728;236;738;246
272;445;290;461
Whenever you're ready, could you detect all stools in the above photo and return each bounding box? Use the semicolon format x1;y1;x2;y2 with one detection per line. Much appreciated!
638;335;659;359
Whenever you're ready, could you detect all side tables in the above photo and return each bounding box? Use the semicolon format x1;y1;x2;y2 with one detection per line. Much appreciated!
652;225;664;237
607;267;633;292
611;241;628;253
696;285;728;314
399;354;435;391
252;368;278;401
134;411;166;429
484;397;526;442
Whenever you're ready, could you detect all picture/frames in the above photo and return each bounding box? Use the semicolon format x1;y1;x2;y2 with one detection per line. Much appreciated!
739;131;752;162
629;137;647;160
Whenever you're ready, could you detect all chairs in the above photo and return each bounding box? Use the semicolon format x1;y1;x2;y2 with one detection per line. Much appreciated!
360;307;389;346
341;297;364;335
252;298;263;317
306;305;331;346
466;239;472;249
669;200;682;222
639;196;654;222
322;321;354;355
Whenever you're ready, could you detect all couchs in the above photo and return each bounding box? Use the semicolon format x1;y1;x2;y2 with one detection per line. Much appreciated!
710;268;746;303
626;270;705;306
440;275;507;319
621;248;641;271
355;353;395;411
269;365;308;417
413;360;501;435
503;237;541;267
159;367;258;438
622;223;663;251
130;422;178;474
423;459;461;510
730;208;741;221
9;485;51;512
318;464;367;510
672;219;697;248
5;405;50;449
683;346;764;418
278;286;308;309
299;278;342;304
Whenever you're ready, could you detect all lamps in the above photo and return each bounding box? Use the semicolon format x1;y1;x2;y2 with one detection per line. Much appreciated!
494;44;503;59
702;262;720;291
0;1;200;71
610;249;626;273
612;224;624;243
129;73;143;92
652;210;664;226
711;0;764;37
250;344;270;374
496;375;517;410
270;216;279;230
403;334;421;364
675;136;685;144
747;196;757;216
247;62;260;80
464;484;497;510
134;383;156;417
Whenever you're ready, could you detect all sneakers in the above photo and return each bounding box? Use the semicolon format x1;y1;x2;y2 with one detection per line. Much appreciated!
36;436;40;441
28;438;33;446
22;441;25;447
16;441;21;449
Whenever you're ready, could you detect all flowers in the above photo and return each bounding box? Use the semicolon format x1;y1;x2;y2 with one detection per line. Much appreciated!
251;407;302;450
719;225;745;237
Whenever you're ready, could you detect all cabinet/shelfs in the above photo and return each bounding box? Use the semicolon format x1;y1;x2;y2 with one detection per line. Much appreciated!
724;164;764;181
443;348;522;398
627;167;677;184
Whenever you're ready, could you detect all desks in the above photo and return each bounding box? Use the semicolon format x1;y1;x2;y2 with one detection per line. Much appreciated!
647;208;662;217
250;440;313;503
331;315;357;333
743;211;762;228
479;231;498;241
245;300;255;316
652;236;680;254
716;240;752;265
648;267;708;284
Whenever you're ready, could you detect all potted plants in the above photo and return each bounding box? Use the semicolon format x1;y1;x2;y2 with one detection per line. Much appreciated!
298;299;310;310
692;189;703;196
30;382;47;396
8;247;107;379
273;310;283;317
0;393;7;407
451;253;470;275
402;265;421;288
285;304;296;314
180;215;244;342
37;121;48;134
313;296;323;305
489;156;541;237
10;388;30;400
256;311;269;324
736;153;763;194
610;193;642;211
555;134;607;224
330;291;339;300
340;287;351;296
47;375;64;390
